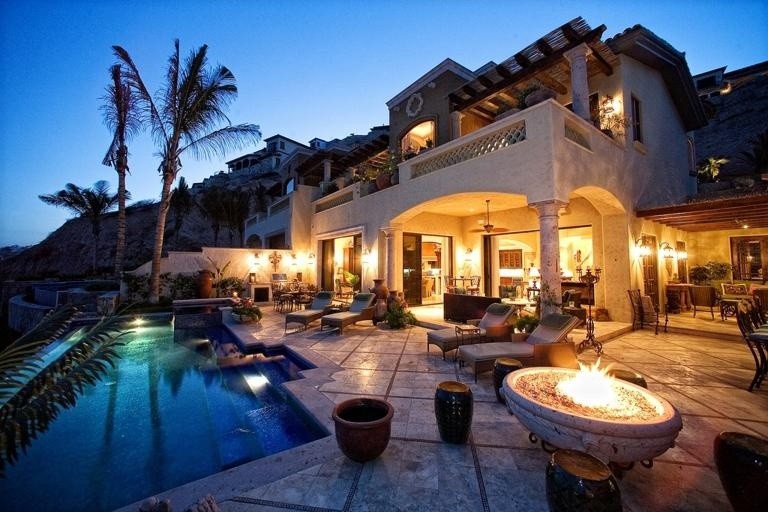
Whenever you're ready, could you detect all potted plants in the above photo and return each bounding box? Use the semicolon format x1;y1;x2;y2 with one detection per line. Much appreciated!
389;155;403;187
374;161;391;189
355;164;379;192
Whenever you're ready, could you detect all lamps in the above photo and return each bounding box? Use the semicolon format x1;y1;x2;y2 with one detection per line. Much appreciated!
252;253;262;267
528;265;541;290
573;262;605;359
657;239;676;256
308;253;315;267
360;248;372;264
632;236;652;258
675;246;689;263
601;98;614;114
290;253;298;266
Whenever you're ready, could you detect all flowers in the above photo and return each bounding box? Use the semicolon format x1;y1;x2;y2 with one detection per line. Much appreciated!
231;297;262;323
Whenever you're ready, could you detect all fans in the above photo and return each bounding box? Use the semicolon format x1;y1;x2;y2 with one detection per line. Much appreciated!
466;198;512;235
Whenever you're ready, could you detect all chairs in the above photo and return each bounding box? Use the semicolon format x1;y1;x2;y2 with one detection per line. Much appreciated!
454;312;584;386
270;275;353;313
624;267;767;395
421;272;481;303
284;289;334;332
319;292;380;336
426;302;519;362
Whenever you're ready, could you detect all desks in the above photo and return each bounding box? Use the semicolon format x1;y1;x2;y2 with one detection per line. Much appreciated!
526;287;539;303
454;323;480;368
513;279;530;300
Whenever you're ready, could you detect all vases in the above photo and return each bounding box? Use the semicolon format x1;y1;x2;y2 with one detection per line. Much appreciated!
331;395;396;465
609;364;645;385
194;268;212;297
493;356;524;405
432;380;476;447
544;445;623;511
708;426;765;512
231;311;259;325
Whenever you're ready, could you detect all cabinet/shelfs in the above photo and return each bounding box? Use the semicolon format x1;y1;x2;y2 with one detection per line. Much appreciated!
499;276;522;285
498;250;523;269
